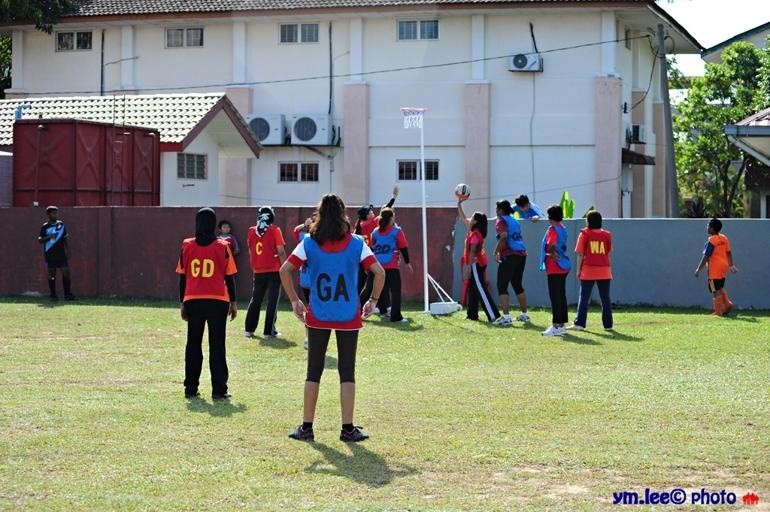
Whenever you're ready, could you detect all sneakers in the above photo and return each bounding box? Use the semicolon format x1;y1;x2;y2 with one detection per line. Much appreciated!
288;426;315;441
340;427;370;442
572;323;584;331
374;306;408;323
64;293;80;301
514;314;529;321
243;330;280;338
541;324;568;337
492;316;512;326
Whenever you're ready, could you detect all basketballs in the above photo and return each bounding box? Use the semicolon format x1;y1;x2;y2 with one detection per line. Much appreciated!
456;183;470;195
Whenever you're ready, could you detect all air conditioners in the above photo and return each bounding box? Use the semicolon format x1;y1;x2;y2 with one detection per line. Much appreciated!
631;124;645;143
246;114;285;146
510;54;541;72
291;113;334;147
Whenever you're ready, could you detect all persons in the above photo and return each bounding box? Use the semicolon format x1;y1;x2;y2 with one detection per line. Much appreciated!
694;218;738;316
454;191;613;336
175;185;415;442
38;206;81;301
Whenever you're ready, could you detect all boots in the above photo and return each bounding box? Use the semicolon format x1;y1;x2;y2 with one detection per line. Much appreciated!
712;294;723;315
720;288;732;313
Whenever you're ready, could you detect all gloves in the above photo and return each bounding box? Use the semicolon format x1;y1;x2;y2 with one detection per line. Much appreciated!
729;265;737;274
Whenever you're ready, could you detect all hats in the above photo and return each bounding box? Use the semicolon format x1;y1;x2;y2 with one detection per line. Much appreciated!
496;200;515;214
358;205;373;219
